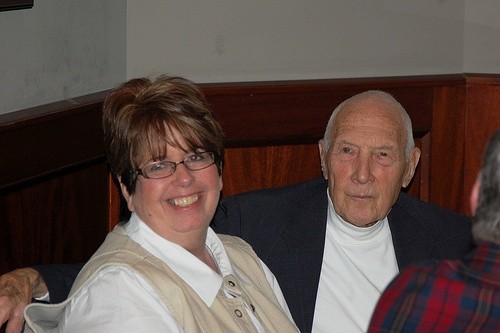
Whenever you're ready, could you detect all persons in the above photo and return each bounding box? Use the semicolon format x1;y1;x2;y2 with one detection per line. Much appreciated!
0;90;476;333
367;128;500;333
24;75;301;333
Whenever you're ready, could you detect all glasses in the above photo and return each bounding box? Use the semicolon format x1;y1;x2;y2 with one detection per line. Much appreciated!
132;150;218;180
